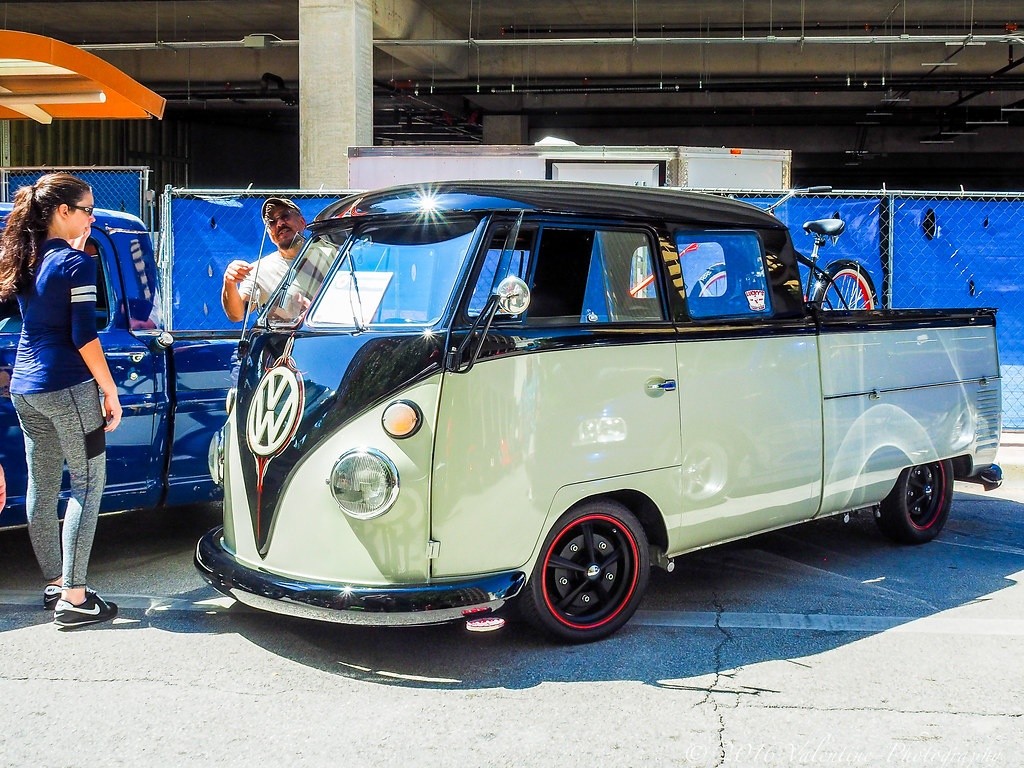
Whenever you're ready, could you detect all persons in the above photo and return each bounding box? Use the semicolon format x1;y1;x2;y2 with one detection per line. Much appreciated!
220;197;339;323
0;172;123;628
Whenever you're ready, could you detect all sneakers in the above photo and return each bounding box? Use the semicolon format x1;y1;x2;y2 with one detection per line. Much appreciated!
53;586;119;627
44;585;63;610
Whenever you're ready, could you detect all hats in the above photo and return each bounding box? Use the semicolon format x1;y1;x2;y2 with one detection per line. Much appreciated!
261;197;302;227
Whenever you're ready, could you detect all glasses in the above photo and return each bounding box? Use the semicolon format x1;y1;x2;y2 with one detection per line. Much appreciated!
58;203;93;216
267;209;300;227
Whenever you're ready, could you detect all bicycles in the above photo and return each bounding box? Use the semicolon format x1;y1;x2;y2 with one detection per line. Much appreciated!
689;180;881;315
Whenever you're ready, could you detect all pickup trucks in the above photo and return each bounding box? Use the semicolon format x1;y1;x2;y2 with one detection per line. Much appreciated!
0;201;254;531
190;179;1007;645
